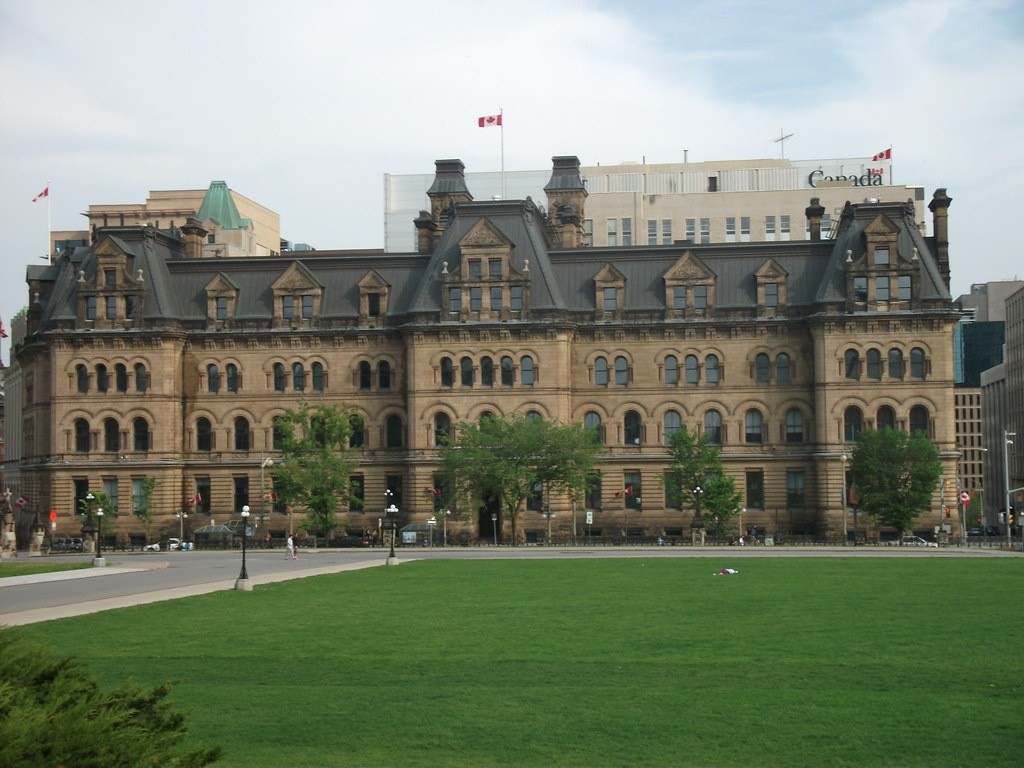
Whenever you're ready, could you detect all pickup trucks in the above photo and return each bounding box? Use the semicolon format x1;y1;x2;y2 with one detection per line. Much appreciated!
881;535;938;548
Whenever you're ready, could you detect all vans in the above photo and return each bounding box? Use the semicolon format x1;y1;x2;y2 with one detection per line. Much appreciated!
51;538;82;551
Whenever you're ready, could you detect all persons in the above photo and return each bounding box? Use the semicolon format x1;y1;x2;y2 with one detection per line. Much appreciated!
366;528;378;548
748;524;759;546
3;488;13;513
177;541;193;551
728;535;745;546
284;532;300;560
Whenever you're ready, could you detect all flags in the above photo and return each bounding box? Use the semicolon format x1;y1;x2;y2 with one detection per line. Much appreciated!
478;114;503;127
615;486;632;497
425;487;441;497
32;186;49;203
873;148;892;161
264;491;277;501
184;494;202;506
957;490;971;508
12;495;29;509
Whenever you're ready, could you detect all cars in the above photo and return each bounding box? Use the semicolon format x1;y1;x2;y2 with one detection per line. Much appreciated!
316;536;372;548
143;537;180;553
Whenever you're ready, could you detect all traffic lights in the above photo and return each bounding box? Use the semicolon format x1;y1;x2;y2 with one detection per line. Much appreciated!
1009;515;1013;523
945;509;951;518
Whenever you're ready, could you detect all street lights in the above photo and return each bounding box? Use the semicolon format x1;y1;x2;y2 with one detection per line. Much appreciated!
444;509;451;546
386;504;399;558
839;453;847;535
95;508;103;557
542;510;557;543
238;505;249;579
690;486;706;529
80;493;98;533
384;489;394;520
1003;440;1012;547
176;511;188;542
260;457;274;549
